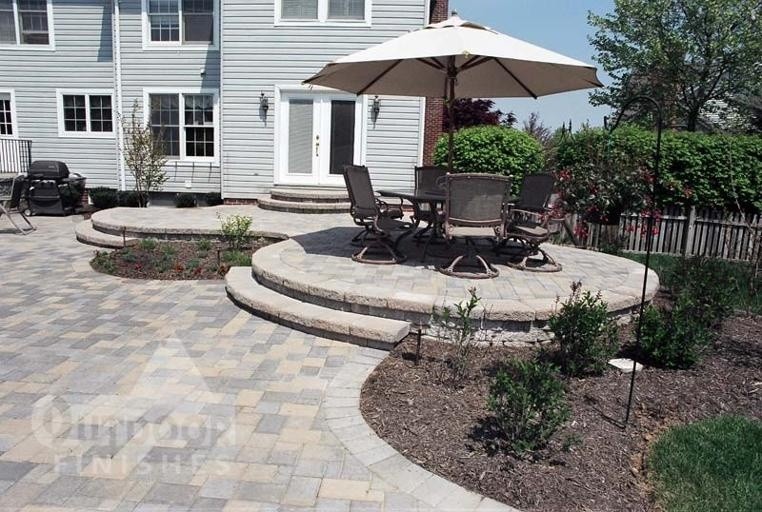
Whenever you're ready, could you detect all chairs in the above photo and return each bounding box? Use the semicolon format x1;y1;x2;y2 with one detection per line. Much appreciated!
339;162;564;278
0;174;38;236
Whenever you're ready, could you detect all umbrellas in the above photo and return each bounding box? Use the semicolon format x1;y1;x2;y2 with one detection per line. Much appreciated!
300;6;604;259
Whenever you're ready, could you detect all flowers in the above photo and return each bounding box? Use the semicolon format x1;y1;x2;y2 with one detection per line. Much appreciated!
545;147;693;239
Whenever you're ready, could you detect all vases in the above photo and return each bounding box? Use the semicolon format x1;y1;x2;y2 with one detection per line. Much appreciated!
583;203;620;226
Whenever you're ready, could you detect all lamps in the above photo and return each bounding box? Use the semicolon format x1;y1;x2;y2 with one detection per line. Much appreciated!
372;94;384;126
258;91;270;118
